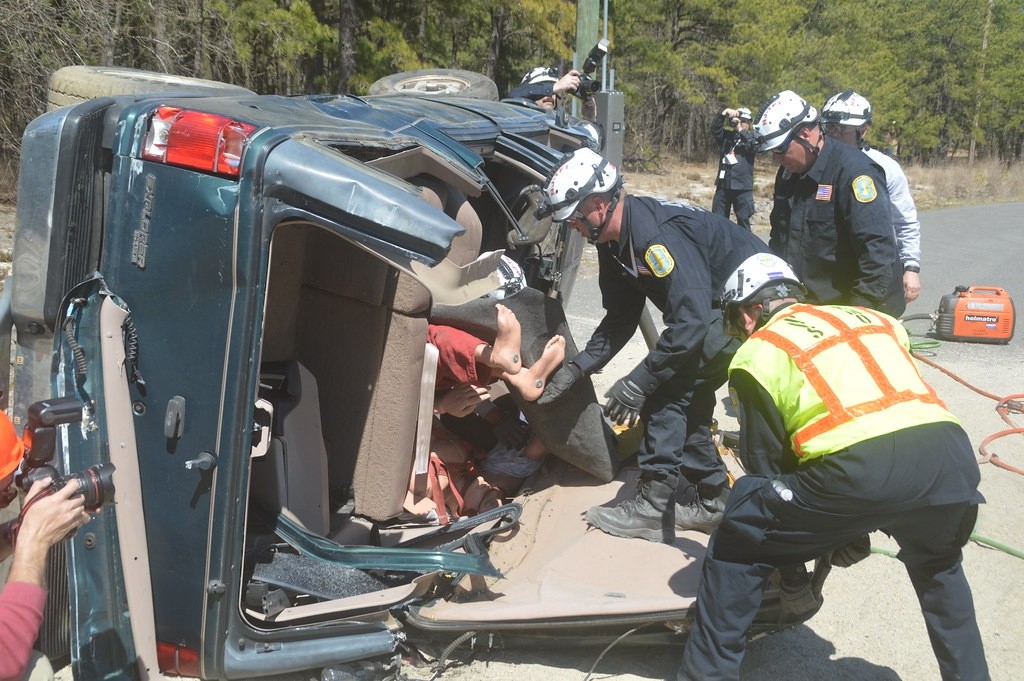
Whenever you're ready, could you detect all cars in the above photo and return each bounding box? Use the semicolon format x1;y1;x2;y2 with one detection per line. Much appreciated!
0;60;825;680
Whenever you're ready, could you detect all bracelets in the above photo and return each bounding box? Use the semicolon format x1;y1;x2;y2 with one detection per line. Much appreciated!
904;266;921;273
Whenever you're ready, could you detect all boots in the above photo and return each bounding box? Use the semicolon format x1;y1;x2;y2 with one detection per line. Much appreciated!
586;473;679;544
675;483;731;535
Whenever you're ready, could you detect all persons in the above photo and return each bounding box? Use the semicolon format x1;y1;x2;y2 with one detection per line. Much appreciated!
675;253;995;681
0;64;923;681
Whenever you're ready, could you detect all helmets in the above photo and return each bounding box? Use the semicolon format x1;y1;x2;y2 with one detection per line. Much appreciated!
820;89;873;125
719;253;808;341
543;147;619;223
753;89;818;153
0;408;24;481
521;66;560;87
736;107;751;120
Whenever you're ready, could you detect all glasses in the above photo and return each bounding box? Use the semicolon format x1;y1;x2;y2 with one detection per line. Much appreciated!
772;123;807;154
565;198;585;225
826;122;861;134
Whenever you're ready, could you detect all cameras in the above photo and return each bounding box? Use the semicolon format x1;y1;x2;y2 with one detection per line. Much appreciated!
729;111;742;127
15;396;118;540
566;43;608;100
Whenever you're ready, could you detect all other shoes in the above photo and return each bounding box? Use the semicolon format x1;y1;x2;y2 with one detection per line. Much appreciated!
482;446;540;478
